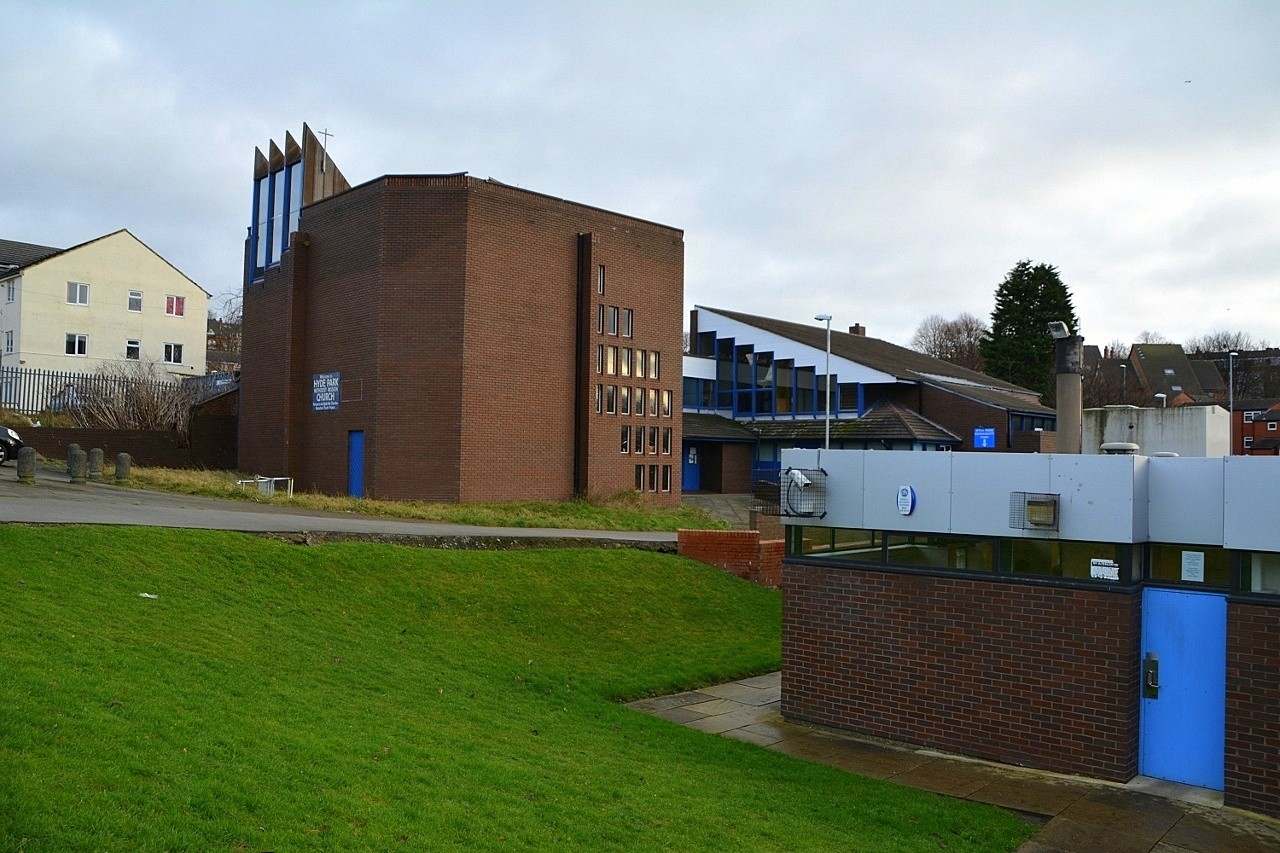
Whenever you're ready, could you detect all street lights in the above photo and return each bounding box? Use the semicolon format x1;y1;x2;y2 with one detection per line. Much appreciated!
1120;364;1129;405
1229;352;1239;457
1155;394;1169;408
813;314;833;451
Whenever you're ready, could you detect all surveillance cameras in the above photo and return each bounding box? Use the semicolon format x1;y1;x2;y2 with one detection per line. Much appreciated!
789;470;811;491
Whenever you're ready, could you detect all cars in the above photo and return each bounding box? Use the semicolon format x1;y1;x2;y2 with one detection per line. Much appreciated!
0;426;27;466
